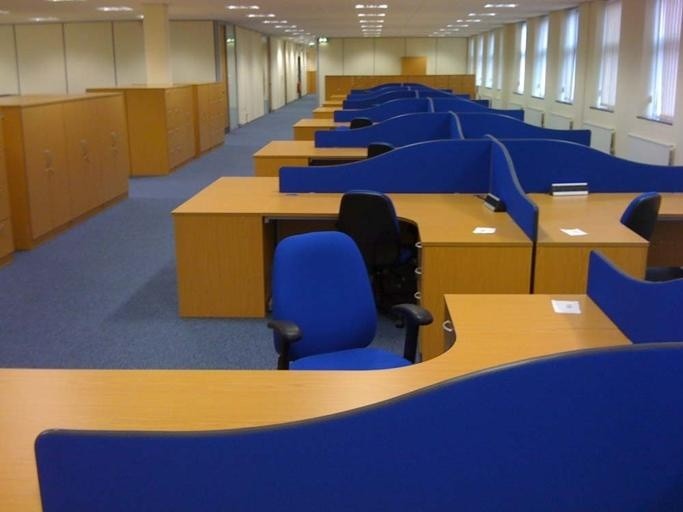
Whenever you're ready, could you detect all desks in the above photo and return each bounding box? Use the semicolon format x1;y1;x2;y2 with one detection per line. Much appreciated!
0;295;683;512
174;172;683;317
251;84;593;175
0;92;129;264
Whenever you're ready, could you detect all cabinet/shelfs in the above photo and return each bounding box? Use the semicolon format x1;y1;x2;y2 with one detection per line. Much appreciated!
322;74;477;102
132;84;228;177
